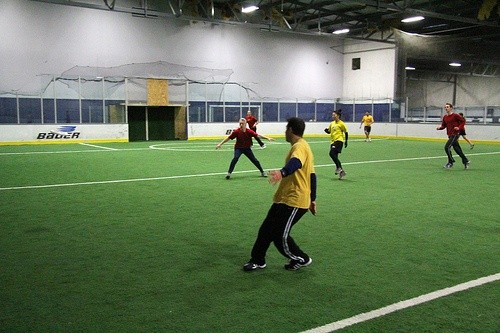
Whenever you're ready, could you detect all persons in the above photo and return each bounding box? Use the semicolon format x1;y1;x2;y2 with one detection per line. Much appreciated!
459;113;475;150
437;103;470;169
216;118;276;179
246;111;266;149
243;117;317;271
360;111;374;142
324;109;349;180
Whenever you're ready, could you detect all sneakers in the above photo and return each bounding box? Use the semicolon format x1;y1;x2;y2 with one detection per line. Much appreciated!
260;145;266;149
443;163;453;169
470;144;474;149
339;170;347;180
243;258;266;270
335;168;342;174
285;257;312;271
464;160;470;169
226;174;230;179
261;172;267;176
